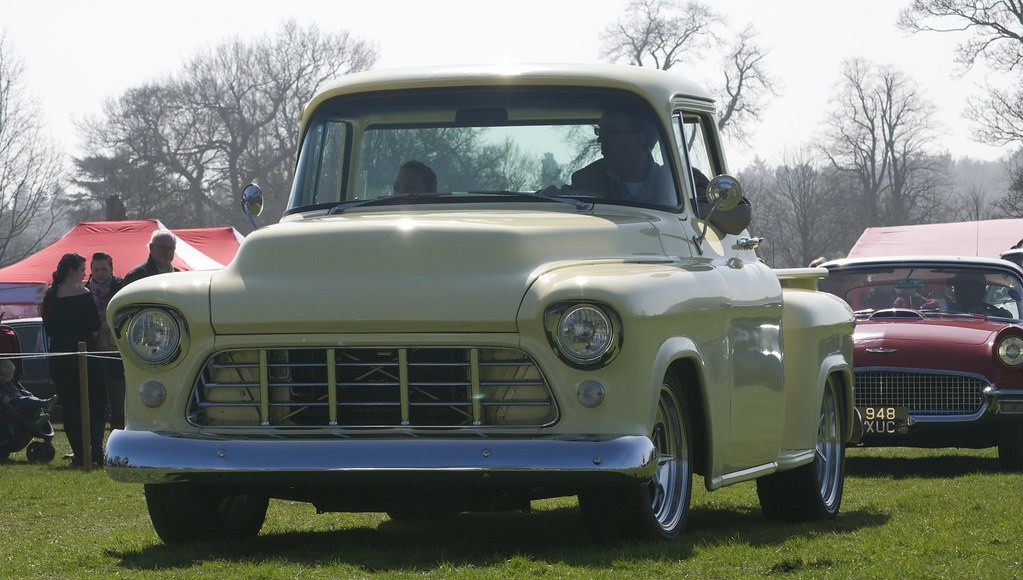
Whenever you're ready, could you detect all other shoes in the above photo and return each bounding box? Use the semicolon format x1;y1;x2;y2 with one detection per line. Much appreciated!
72;447;103;466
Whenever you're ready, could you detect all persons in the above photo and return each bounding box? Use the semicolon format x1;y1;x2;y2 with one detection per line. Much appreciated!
41;253;104;471
390;160;437;193
542;105;751;235
120;229;178;286
945;272;1013;318
0;359;57;428
848;284;954;312
85;253;123;468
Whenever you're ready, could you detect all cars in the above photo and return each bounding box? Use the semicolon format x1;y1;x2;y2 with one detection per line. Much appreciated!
107;68;855;549
809;256;1019;466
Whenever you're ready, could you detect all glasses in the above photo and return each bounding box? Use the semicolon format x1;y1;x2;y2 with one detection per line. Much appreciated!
594;128;641;138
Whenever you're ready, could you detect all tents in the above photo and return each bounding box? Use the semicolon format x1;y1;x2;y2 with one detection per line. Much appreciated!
170;227;247;267
847;218;1023;260
0;220;223;306
